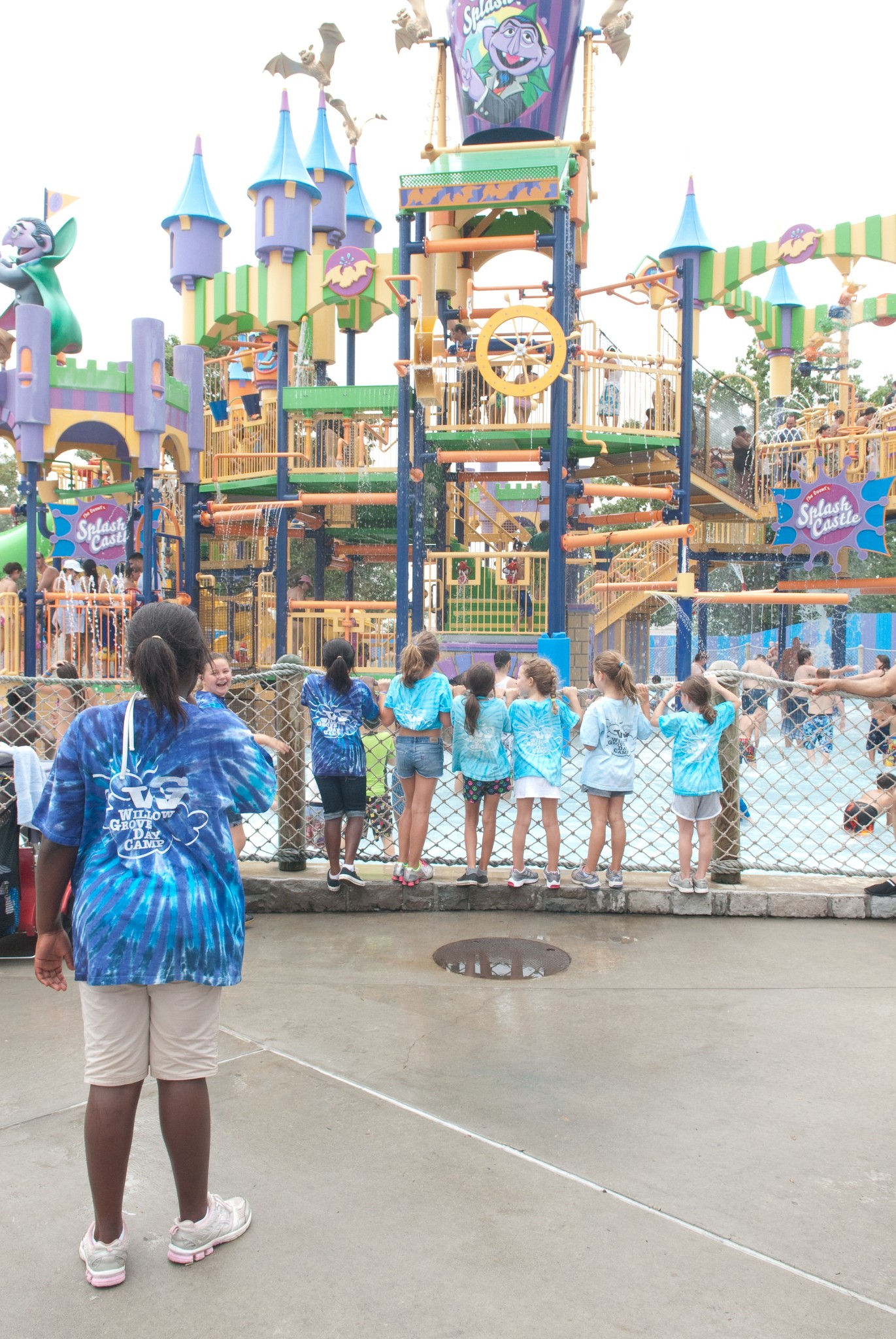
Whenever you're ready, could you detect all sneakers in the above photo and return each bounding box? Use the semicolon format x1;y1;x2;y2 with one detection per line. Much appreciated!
605;866;623;889
543;864;560;889
327;866;341;891
167;1193;252;1266
508;866;538;887
667;873;709;894
339;867;365;888
77;1218;129;1289
570;866;600;891
455;873;489;887
393;860;433;886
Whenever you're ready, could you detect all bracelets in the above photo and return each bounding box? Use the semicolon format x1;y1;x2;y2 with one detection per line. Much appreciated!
379;691;387;695
662;699;668;707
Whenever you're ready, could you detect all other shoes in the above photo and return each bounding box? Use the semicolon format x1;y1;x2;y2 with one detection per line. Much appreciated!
865;879;896;896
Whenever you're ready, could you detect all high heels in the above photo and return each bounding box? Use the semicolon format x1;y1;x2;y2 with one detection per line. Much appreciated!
245;913;253;924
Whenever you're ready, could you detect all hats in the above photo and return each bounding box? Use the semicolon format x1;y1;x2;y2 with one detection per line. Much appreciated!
64;559;85;573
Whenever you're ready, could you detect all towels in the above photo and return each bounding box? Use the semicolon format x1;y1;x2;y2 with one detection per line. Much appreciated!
12;742;50;834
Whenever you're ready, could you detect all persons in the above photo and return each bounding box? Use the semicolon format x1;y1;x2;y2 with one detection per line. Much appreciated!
216;324;896;506
490;651;524;800
525;520;550;599
357;643;377;667
384;647;394;660
340;717;396;860
36;662;99;754
841;773;896;840
651;673;741;894
803;663;896;895
876;703;896;766
766;637;808;718
585;676;603;706
197;652;290;926
260;632;278;665
649;675;664;713
571;650;650;890
35;601;273;1288
838;654;892;720
448;663;510;889
866;698;890;766
510;559;539;636
799;668;845;764
504;656;583;889
691;652;707;675
0;687;54;760
782;649;855;748
739;706;767;770
285;575;312;656
0;550;161;679
378;629;454;888
305;637;381;892
739;654;780;734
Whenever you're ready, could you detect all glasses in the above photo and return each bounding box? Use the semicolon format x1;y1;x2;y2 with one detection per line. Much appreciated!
36;556;42;559
70;570;79;574
